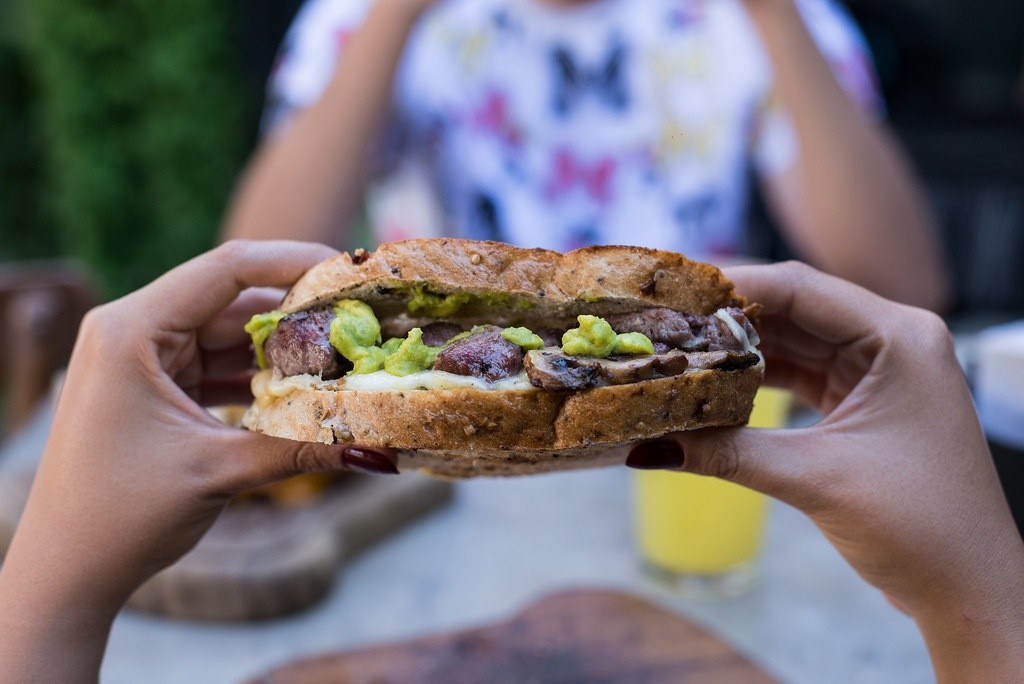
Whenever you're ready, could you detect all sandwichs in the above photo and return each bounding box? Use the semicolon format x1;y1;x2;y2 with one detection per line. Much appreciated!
242;239;765;480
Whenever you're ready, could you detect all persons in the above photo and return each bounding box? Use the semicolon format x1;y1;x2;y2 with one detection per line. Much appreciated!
2;51;98;455
221;1;950;314
1;238;1024;683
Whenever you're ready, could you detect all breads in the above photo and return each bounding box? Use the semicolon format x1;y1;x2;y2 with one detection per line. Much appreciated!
121;469;452;613
